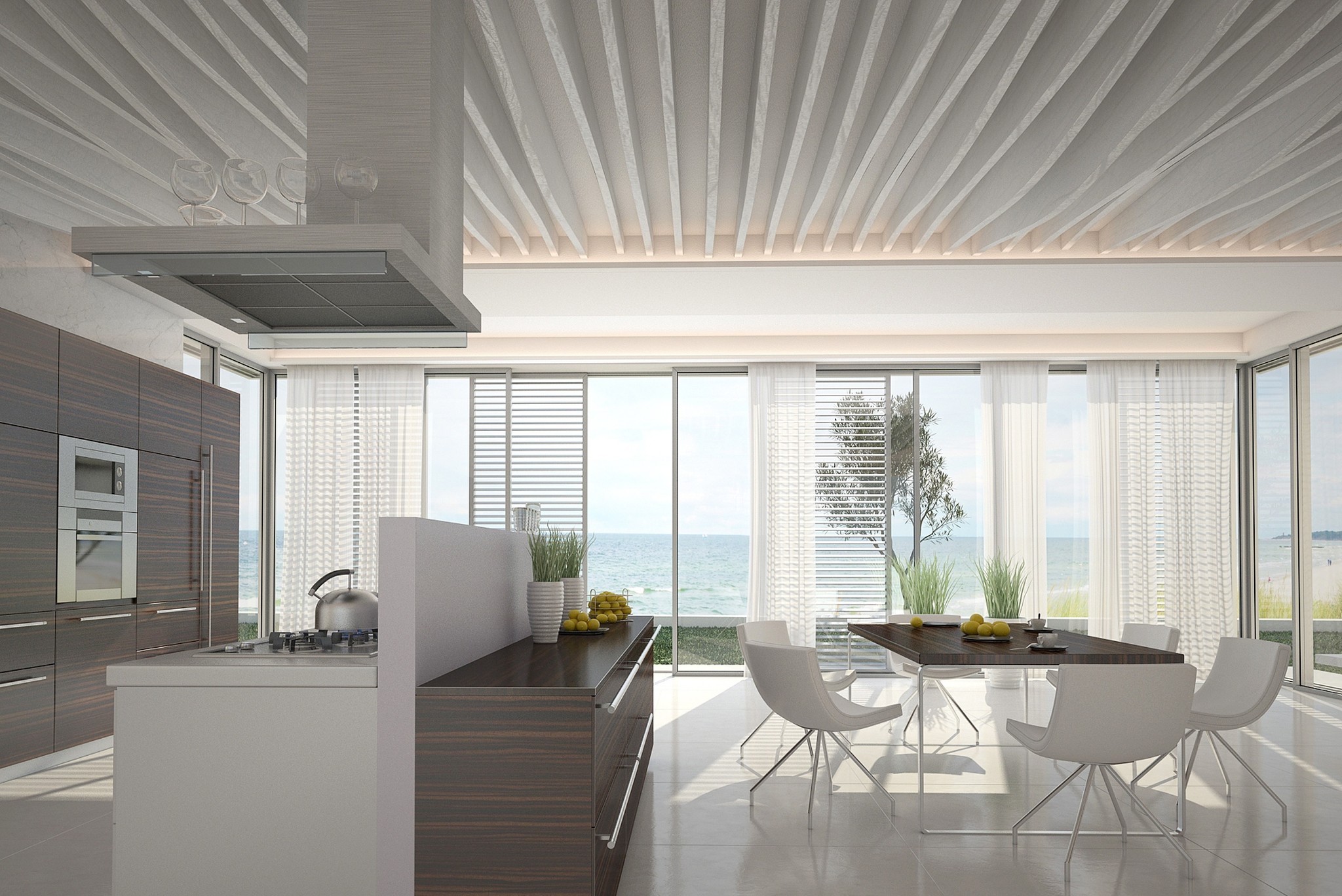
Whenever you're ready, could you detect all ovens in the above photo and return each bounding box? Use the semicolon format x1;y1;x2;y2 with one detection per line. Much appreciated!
56;434;139;603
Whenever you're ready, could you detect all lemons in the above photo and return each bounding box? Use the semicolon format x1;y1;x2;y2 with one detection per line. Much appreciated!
911;617;923;628
563;610;600;631
959;614;1010;636
587;592;631;623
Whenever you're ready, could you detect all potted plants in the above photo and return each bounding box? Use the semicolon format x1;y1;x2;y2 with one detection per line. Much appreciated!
967;552;1035;690
520;521;597;644
890;551;966;676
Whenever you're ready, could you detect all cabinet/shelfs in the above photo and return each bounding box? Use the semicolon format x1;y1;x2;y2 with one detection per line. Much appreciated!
414;616;654;896
0;305;240;773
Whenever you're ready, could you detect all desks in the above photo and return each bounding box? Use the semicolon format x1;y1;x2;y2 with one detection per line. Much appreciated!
847;621;1184;834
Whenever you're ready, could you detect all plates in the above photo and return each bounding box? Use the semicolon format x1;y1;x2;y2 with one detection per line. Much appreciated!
1022;628;1054;631
1030;645;1069;649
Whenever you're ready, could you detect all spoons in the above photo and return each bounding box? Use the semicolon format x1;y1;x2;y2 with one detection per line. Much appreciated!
1010;643;1039;651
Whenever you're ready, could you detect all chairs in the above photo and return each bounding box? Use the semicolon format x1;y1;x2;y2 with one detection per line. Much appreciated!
743;641;902;815
734;620;858;759
888;655;982;742
1005;660;1196;866
1044;625;1180;782
1128;637;1292;832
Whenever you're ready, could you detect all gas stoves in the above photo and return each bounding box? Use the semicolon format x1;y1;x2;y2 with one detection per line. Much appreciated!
105;626;379;688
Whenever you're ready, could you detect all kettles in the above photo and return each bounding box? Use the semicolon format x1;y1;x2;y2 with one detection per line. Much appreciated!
308;571;381;632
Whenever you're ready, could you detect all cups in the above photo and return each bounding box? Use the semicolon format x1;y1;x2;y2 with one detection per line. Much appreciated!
1036;632;1059;647
1028;619;1046;630
